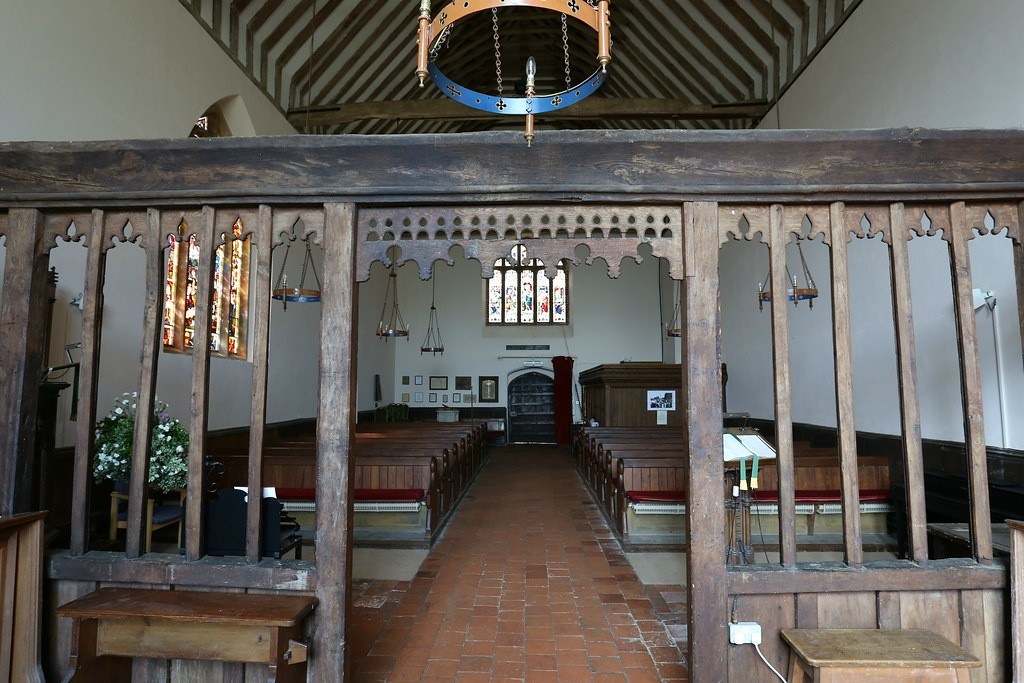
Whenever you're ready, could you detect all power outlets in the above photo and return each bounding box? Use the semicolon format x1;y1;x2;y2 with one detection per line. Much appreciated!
728;621;762;645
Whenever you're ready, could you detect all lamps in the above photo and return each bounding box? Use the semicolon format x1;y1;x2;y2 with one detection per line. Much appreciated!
415;0;612;149
421;262;444;357
272;220;322;313
375;238;410;342
664;280;683;340
757;235;819;313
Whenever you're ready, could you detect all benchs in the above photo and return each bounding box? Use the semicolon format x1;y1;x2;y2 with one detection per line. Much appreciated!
572;424;898;549
193;420;489;551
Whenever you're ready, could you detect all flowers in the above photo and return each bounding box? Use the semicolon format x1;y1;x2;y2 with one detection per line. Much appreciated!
93;391;189;495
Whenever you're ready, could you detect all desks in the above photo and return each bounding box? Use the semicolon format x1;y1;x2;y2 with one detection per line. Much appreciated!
781;628;983;683
53;587;319;683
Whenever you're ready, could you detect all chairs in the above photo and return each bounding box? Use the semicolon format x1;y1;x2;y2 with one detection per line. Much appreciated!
180;456;304;562
110;477;187;554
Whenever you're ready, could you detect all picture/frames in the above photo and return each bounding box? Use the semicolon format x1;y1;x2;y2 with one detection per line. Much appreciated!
402;376;500;403
